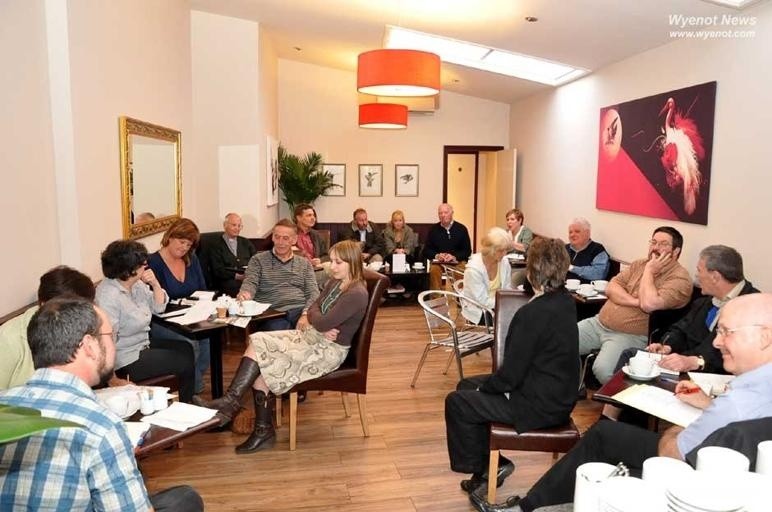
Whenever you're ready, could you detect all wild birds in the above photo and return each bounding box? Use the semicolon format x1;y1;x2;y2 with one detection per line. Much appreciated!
656;97;706;217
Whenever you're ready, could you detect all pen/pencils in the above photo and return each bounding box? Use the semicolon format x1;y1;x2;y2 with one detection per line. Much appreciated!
127;374;129;382
674;388;701;396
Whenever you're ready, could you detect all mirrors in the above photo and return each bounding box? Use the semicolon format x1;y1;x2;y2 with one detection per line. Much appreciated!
116;115;184;240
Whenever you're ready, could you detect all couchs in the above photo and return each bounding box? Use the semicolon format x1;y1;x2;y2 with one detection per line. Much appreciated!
308;221;468;301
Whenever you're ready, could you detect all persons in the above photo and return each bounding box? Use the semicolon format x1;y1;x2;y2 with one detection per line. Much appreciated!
90;202;368;455
445;237;580;497
0;295;205;512
469;294;772;512
0;263;95;391
578;228;694;384
338;205;612;328
597;243;762;429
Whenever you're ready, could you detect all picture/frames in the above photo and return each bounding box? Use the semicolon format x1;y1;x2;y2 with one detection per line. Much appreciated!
394;164;420;196
358;164;384;197
317;163;346;197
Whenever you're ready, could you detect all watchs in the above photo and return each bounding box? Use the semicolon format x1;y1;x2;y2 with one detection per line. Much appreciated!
695;356;705;372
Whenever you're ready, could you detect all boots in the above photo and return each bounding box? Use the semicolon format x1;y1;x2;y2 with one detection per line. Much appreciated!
192;356;261;427
235;387;275;454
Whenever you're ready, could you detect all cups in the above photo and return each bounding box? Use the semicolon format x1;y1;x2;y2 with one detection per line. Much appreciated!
752;440;771;474
414;263;422;267
640;457;695;480
140;399;155;415
577;285;593;294
242;300;258;313
629;357;654;375
567;280;580;288
106;396;130;414
574;461;622;512
695;446;749;479
591;280;608;289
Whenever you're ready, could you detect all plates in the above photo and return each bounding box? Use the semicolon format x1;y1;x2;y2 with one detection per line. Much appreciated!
236;310;263;316
412;266;425;270
564;285;577;291
598;473;661;512
121;404;138;420
594;289;606;292
621;365;660;380
577;291;597;296
666;475;746;512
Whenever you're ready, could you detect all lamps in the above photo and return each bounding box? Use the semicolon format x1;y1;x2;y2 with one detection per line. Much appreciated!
356;49;441;98
357;103;408;130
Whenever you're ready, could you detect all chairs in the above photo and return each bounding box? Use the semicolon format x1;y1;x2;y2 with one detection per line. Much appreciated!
274;269;392;450
487;291;580;506
197;232;239;290
409;290;496;389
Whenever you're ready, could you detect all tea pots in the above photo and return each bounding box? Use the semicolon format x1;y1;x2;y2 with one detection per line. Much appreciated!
225;298;241;314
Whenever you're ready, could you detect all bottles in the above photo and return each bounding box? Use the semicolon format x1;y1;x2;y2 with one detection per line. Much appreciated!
385;263;390;271
215;307;227;317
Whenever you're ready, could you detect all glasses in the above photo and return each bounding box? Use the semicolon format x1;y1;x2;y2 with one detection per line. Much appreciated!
649;240;672;248
90;331;120;344
716;326;767;336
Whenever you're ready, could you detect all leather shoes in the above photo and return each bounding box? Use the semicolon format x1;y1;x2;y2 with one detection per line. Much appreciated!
461;456;523;512
